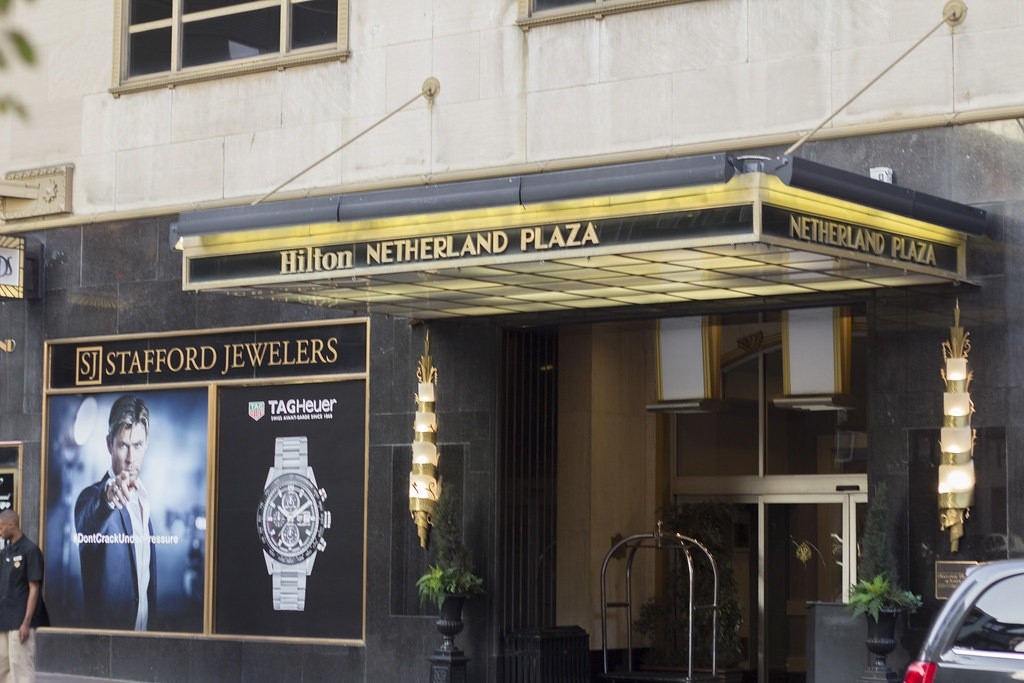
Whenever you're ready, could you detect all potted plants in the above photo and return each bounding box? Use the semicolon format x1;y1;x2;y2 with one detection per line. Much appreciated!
848;479;923;666
632;499;749;682
415;481;487;649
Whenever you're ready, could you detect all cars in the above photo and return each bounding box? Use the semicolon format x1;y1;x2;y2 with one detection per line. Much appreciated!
978;534;1007;553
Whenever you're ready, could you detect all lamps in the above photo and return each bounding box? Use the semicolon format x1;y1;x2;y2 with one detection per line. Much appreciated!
937;294;977;553
409;319;442;550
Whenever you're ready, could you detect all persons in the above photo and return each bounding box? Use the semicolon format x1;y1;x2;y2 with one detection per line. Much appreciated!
74;394;157;631
0;510;50;683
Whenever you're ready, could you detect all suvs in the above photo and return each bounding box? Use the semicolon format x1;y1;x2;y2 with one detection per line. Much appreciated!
902;559;1024;683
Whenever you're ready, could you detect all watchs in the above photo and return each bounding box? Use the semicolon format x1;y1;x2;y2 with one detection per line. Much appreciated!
256;436;331;612
107;482;112;488
